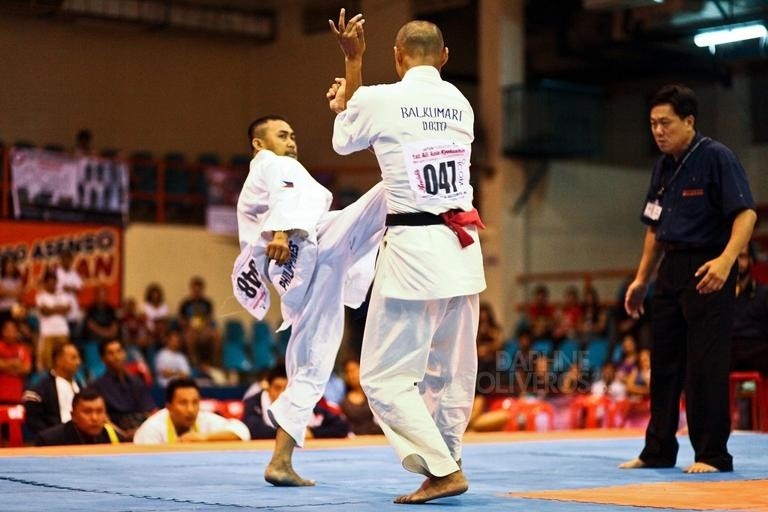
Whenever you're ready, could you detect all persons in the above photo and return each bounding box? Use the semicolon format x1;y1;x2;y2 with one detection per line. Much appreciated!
231;113;387;488
326;7;488;504
618;83;759;474
